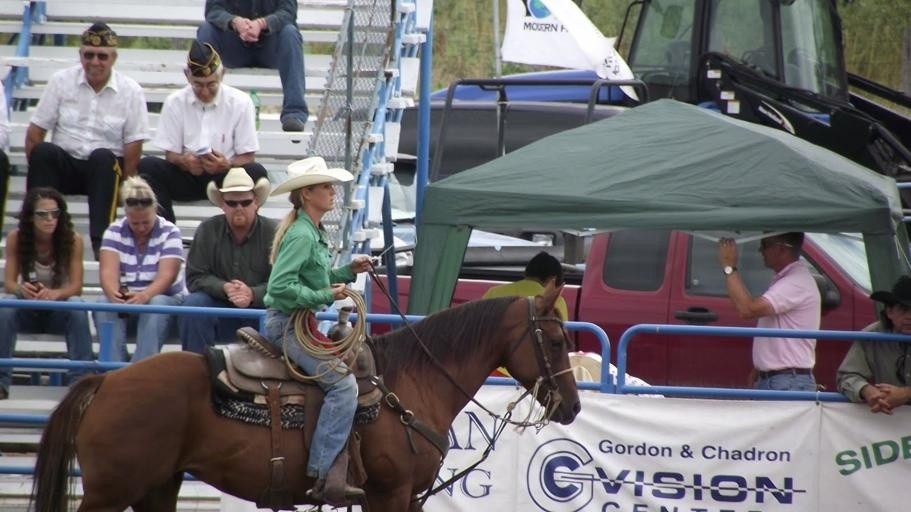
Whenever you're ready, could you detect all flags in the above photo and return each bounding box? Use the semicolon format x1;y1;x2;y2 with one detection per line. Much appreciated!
501;0;639;103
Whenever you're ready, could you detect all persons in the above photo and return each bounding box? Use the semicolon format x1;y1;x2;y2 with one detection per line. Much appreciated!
195;1;309;132
484;252;570;377
0;79;12;236
178;168;278;351
0;187;90;400
26;22;148;261
263;157;386;501
90;176;188;363
138;40;269;227
718;232;821;392
837;275;911;415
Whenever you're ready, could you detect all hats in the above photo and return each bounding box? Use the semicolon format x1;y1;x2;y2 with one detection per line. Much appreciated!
81;21;117;47
269;156;354;196
187;40;222;76
206;167;271;210
869;276;911;311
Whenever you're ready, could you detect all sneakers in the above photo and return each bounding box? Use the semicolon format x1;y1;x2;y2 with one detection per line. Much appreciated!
282;117;305;131
305;477;364;498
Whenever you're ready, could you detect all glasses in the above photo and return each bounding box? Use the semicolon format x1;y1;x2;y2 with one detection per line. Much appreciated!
306;184;334;191
896;355;906;384
83;51;109;60
33;208;61;220
223;198;254;207
762;239;781;247
125;197;153;207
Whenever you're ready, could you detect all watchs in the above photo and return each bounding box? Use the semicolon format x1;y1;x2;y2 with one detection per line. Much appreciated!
720;265;738;275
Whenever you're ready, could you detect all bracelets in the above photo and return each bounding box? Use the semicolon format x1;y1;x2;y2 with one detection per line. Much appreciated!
256;18;267;29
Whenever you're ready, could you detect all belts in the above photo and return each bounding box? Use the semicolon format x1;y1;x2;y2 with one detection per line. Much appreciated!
759;368;810;379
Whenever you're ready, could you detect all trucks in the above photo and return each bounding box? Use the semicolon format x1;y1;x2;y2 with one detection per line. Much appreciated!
364;223;878;395
399;2;910;188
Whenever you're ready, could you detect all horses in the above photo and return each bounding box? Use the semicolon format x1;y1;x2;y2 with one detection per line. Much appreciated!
26;278;581;512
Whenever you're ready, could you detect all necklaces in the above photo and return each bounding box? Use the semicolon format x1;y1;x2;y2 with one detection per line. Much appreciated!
37;245;53;259
